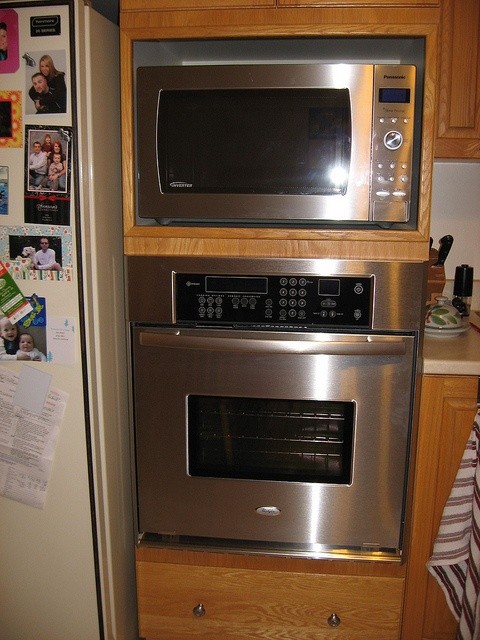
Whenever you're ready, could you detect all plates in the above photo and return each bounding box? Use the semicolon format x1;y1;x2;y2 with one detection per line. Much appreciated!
424;321;471;338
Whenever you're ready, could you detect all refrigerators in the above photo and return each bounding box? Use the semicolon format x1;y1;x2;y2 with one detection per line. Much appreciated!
0;1;138;639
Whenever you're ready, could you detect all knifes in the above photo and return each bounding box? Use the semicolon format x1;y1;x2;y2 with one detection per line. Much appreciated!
430;234;453;269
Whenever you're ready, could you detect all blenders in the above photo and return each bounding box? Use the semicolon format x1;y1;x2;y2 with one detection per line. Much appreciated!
452;264;474;317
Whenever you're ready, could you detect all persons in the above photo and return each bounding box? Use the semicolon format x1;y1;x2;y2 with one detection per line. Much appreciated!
34;237;62;270
28;55;66;110
17;333;47;361
29;134;66;191
31;73;65;114
0;315;30;361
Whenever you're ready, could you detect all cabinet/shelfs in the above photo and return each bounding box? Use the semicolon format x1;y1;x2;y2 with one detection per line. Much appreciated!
121;0;434;264
136;547;408;639
428;0;480;163
404;372;480;640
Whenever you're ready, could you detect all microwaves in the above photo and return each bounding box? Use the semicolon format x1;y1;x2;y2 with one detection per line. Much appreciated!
131;37;428;230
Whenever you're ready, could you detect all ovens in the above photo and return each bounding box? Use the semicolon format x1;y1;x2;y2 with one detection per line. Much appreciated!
125;256;428;563
